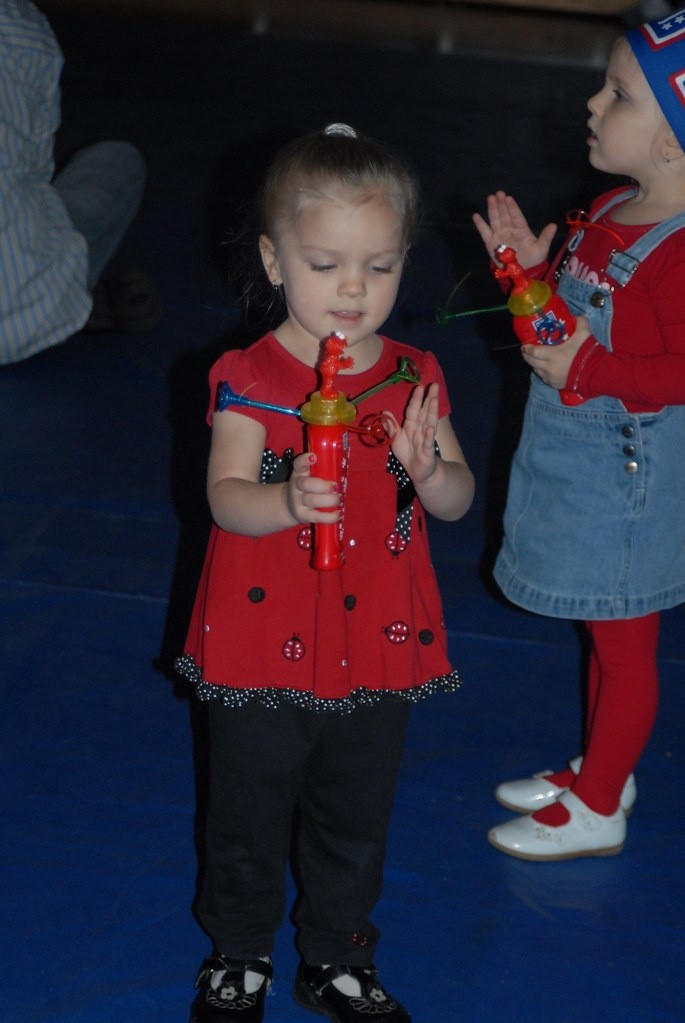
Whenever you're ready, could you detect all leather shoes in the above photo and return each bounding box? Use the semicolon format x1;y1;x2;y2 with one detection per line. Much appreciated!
488;788;628;860
498;756;637;814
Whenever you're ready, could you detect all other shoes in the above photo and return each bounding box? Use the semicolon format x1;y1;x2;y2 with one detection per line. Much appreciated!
290;955;412;1023
189;953;275;1023
87;268;162;333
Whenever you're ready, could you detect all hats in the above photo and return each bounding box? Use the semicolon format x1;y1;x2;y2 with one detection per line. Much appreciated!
623;10;685;153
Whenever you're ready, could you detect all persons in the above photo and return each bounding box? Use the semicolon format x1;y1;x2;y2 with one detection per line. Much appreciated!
174;121;478;1023
471;5;685;858
0;0;162;366
